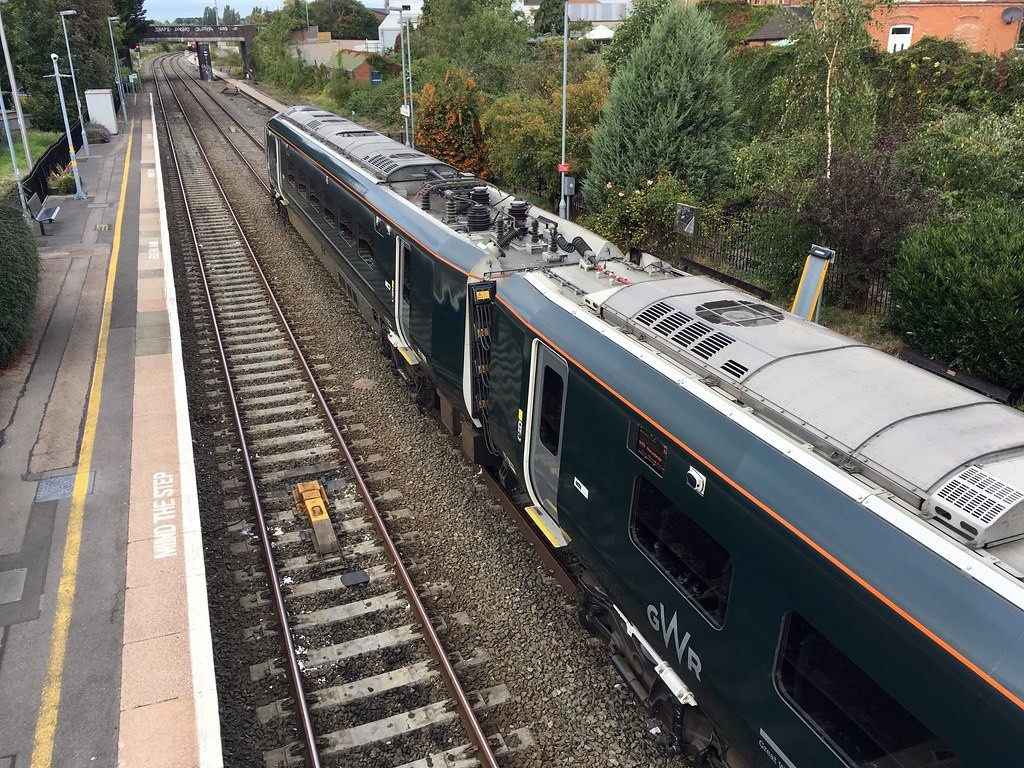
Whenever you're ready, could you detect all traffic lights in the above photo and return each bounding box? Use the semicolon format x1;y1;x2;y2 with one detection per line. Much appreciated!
135;45;140;51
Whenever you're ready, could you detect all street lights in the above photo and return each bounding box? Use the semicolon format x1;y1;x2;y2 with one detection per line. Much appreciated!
59;10;89;158
108;16;128;124
386;5;410;147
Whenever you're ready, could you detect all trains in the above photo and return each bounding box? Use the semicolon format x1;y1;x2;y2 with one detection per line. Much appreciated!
262;107;1024;768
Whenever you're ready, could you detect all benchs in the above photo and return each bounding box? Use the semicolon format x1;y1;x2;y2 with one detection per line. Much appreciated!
27;192;60;236
